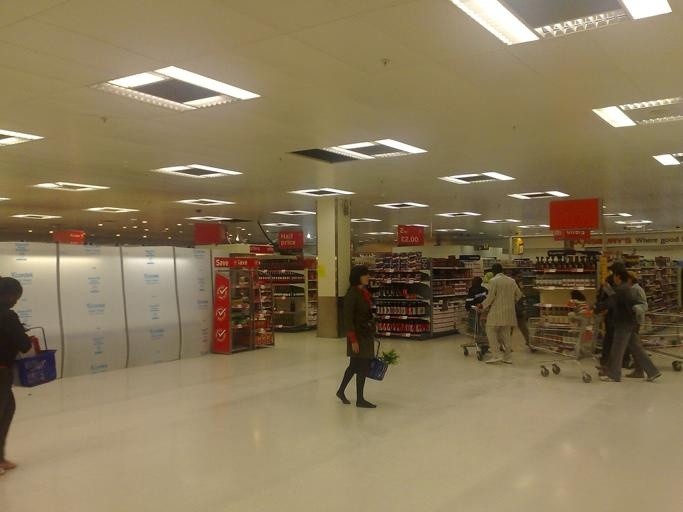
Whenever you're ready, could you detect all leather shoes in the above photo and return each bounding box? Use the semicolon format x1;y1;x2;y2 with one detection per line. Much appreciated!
357;400;377;408
337;389;350;404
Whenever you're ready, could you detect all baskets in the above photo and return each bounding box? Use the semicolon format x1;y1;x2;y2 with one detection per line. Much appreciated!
367;338;388;381
13;326;56;387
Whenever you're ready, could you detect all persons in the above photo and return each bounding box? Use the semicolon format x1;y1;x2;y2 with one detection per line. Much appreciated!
509;268;537;354
464;276;487;312
567;291;588;329
594;263;661;383
476;263;523;364
335;265;377;408
0;277;31;475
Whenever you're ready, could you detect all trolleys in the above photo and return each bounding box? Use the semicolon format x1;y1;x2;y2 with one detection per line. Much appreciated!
524;310;599;383
633;308;682;371
454;301;487;362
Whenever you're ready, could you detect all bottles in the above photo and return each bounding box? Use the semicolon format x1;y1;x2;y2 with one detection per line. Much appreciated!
272;286;304;297
534;255;597;270
255;272;304;283
289;300;294;311
273;303;276;311
374;300;431;332
540;306;580;343
250;317;271;329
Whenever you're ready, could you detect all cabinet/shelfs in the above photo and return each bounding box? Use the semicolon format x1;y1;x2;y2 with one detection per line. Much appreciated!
533;253;682;350
255;270;273;346
352;253;539;341
307;257;317;332
213;258;255;355
258;255;307;333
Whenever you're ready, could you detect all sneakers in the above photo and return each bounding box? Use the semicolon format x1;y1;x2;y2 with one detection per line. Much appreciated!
486;355;502;361
596;364;661;383
